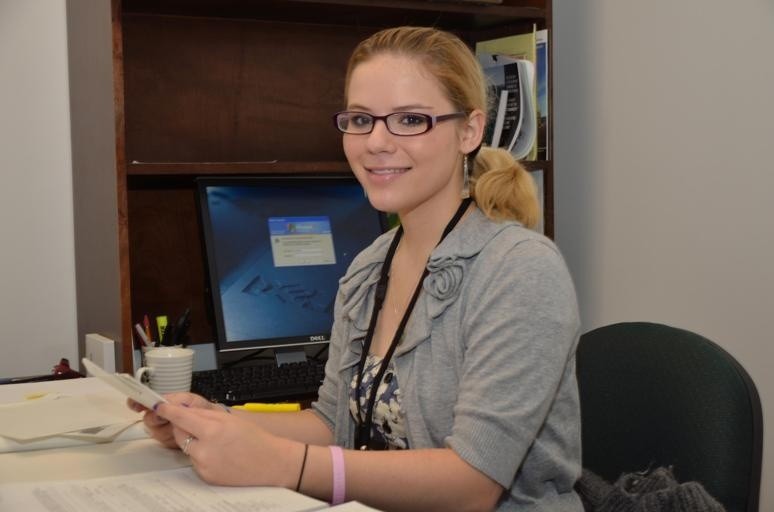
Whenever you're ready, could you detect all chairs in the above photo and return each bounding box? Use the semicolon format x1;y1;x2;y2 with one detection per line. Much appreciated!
577;319;765;511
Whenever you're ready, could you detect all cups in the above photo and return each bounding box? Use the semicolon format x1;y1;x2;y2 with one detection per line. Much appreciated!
140;343;184;383
135;347;194;394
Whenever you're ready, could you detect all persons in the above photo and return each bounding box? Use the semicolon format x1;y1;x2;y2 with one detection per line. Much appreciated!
123;27;587;512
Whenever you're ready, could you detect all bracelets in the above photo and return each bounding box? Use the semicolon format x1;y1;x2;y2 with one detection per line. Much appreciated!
294;443;308;493
327;443;346;506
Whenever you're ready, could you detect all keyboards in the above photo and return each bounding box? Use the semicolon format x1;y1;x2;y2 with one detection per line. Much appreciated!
191;357;326;406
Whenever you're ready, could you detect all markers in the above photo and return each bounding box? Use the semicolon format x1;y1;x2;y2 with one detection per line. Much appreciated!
227;403;301;414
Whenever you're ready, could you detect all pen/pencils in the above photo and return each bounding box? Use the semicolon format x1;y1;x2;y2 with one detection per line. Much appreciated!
136;316;160;347
178;307;192;326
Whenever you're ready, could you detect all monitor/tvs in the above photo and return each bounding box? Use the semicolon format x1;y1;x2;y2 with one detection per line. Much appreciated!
192;174;387;362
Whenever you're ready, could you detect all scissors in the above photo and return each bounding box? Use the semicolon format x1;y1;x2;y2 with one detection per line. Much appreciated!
163;322;191;346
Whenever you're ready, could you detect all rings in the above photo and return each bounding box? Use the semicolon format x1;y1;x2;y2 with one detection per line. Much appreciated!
181;435;194;455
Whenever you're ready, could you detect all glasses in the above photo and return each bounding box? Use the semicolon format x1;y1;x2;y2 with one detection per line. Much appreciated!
332;108;470;136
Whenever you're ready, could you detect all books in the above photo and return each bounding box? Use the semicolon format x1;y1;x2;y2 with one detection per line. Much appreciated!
471;24;550;161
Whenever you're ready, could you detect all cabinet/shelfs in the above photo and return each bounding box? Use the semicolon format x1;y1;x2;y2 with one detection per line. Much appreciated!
62;1;558;381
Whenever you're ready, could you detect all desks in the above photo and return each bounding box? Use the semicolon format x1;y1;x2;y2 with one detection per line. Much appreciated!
0;369;365;512
